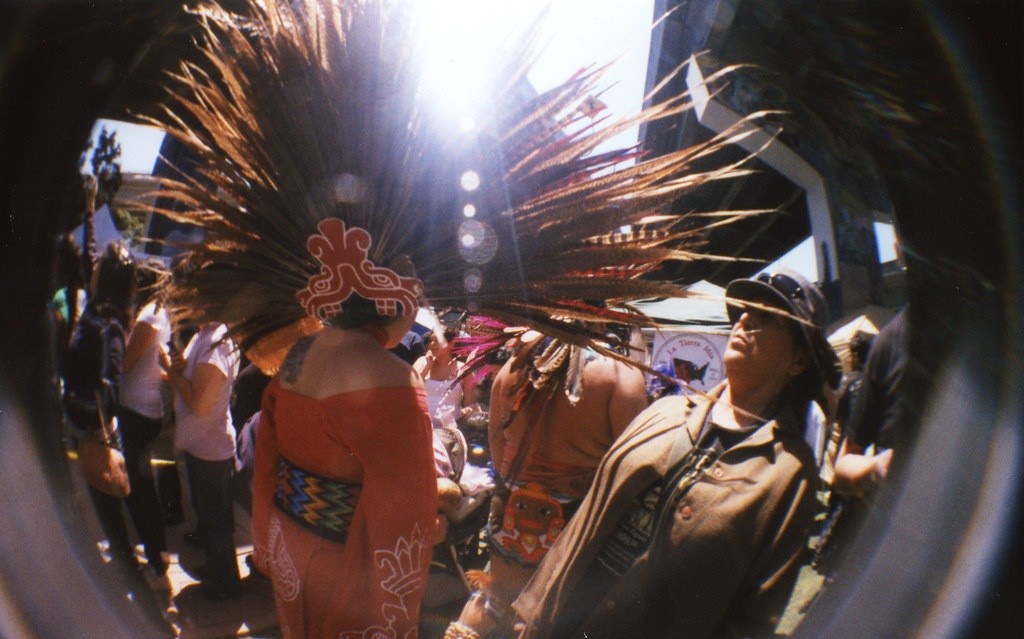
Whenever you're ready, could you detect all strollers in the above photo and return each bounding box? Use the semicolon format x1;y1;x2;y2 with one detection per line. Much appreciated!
419;429;496;571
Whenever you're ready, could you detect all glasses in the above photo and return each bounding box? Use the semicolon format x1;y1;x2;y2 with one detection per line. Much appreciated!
756;272;817;317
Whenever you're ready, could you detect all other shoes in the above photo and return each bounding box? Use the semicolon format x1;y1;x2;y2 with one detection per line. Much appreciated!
136;563;172;592
134;543;170;565
184;532;205;548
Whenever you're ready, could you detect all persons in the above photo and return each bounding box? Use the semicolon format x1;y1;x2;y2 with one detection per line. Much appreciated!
413;330;474;428
832;237;914;527
119;259;173;591
156;311;242;600
254;254;439;638
49;238;88;397
441;304;647;639
64;241;139;569
512;269;827;639
811;331;876;567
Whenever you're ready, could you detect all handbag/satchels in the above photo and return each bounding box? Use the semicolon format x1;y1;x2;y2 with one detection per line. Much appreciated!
485;480;579;566
77;380;133;498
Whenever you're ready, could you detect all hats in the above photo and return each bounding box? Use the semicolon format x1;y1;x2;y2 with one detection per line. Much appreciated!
726;265;843;390
